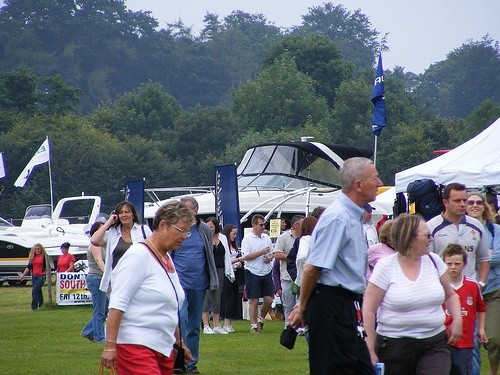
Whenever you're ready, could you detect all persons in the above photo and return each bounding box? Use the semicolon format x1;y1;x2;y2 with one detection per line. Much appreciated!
462;191;500;374
201;215;236;336
240;213;277;336
217;223;247;333
54;241;77;273
439;242;489;374
99;199;196;375
361;213;464;375
424;181;490;374
80;220;111;343
20;242;51;313
91;201;154;302
272;189;500;349
168;194;218;375
285;155;384;374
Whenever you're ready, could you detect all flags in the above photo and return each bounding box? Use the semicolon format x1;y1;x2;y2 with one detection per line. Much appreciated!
13;136;51;188
369;51;387;137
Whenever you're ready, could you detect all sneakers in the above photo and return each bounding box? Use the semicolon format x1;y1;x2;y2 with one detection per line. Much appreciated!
286;325;306;335
204;325;215;334
213;325;228;334
250;325;260;334
257;321;264;333
223;325;235;332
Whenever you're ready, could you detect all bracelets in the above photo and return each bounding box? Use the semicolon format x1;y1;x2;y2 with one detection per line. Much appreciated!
106;339;117;343
102;348;118;352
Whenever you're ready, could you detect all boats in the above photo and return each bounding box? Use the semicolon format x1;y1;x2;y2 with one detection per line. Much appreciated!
0;192;101;271
121;135;374;232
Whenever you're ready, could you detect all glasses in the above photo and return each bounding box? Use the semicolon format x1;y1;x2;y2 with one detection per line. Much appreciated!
170;223;191;237
255;223;267;227
466;200;483;206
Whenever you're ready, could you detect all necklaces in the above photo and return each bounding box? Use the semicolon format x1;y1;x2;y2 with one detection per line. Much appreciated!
211;234;219;248
147;236;175;270
